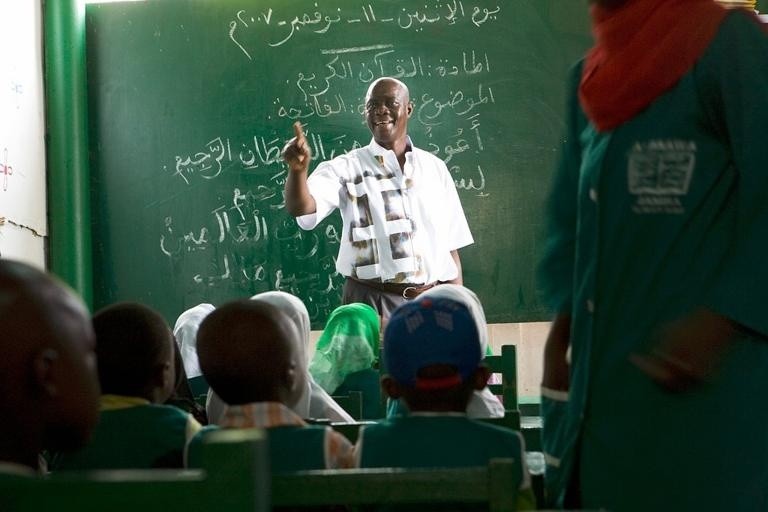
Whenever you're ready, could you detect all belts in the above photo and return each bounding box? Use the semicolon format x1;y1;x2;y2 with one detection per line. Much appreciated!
366;282;438;298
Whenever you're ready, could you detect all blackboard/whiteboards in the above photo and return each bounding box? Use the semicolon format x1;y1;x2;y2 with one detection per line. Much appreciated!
85;0;597;331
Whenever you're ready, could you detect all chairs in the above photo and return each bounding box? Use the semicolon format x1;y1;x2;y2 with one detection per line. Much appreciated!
1;346;521;511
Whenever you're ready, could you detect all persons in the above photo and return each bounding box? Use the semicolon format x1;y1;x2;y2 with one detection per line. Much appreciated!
279;76;475;348
536;0;768;512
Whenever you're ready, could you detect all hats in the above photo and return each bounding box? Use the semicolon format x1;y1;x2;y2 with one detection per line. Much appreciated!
377;297;482;393
